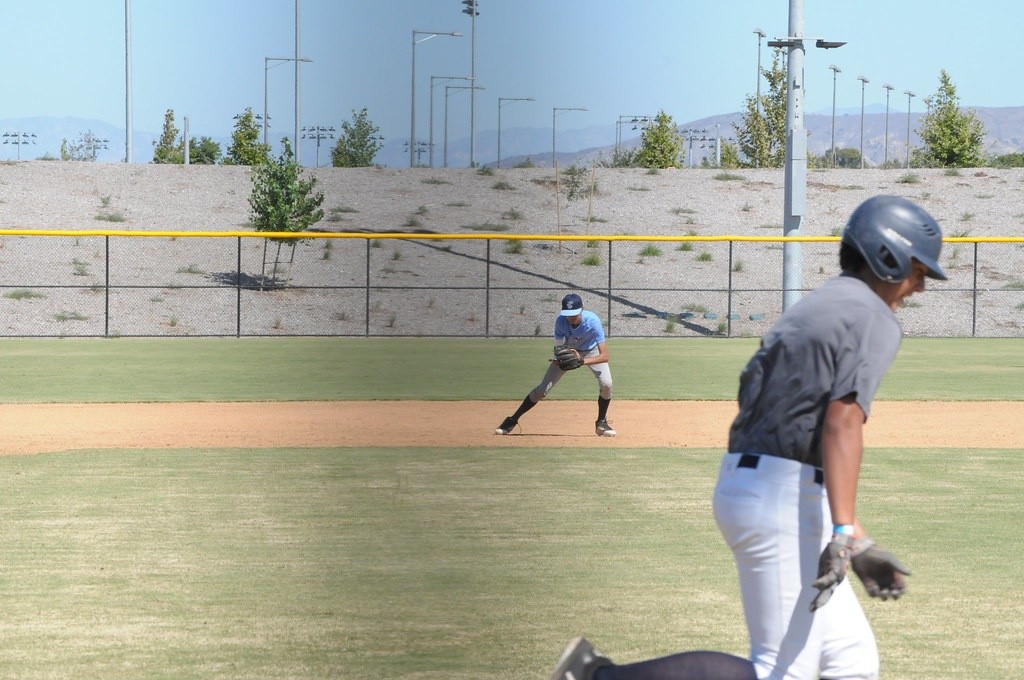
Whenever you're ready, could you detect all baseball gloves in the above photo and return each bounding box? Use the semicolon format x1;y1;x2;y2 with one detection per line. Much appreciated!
554;345;584;371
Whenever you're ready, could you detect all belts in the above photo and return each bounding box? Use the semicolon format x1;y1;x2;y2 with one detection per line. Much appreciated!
737;453;824;485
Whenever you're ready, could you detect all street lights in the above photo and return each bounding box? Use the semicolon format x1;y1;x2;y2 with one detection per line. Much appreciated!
856;74;870;169
78;137;110;162
410;29;466;167
496;96;653;167
752;29;767;170
443;85;486;168
1;131;39;161
265;57;316;144
401;138;436;164
299;125;336;168
829;65;843;167
674;128;710;169
903;89;917;169
428;75;476;168
882;83;895;169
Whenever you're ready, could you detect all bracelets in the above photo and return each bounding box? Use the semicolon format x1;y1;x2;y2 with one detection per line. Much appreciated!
833;524;854;534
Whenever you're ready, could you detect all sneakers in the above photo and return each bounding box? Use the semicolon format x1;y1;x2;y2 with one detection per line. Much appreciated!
547;636;612;680
495;416;519;435
596;419;616;437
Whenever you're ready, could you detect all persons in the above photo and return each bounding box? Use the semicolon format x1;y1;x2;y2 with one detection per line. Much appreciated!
550;195;949;680
495;293;616;437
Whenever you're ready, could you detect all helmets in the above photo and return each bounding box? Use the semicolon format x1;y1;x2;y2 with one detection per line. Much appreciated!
839;196;946;284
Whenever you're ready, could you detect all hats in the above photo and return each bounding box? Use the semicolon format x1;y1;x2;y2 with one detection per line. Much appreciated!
560;293;582;316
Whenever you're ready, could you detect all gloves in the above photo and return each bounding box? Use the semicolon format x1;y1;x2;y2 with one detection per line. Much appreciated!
809;532;857;612
849;537;911;599
